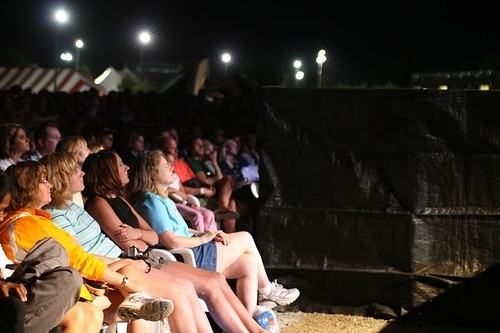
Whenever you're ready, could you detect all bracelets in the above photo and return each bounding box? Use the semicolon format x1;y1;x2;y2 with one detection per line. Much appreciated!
117;276;129;289
138;231;144;239
200;187;204;195
199;236;205;244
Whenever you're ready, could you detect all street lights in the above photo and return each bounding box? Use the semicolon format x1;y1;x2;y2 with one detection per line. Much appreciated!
221;52;232;81
315;49;327;89
137;32;151;79
292;60;304;88
53;7;69;93
74;39;84;74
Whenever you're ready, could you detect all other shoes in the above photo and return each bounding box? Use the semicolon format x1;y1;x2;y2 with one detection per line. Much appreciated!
215;208;239;220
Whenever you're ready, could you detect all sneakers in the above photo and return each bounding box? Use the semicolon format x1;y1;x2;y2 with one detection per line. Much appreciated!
258;278;300;308
116;293;174;322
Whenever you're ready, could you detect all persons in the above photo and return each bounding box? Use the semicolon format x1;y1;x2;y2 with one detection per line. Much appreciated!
81;150;270;333
125;148;301;321
0;151;214;333
0;84;284;234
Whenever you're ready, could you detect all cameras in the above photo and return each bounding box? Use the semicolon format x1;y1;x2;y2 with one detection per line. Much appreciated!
120;247;148;259
